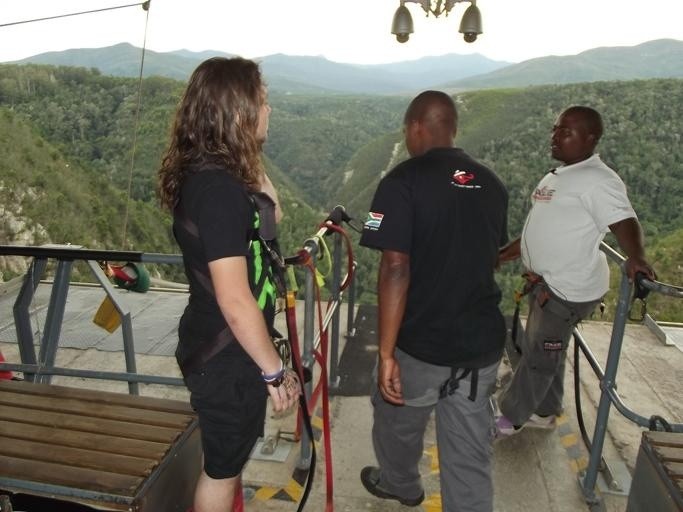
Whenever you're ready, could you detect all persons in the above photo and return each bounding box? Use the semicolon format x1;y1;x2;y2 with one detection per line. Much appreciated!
155;55;304;512
357;89;510;512
490;105;657;438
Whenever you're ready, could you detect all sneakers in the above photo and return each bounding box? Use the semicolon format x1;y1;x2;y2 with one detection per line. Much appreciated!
490;404;558;443
357;465;427;508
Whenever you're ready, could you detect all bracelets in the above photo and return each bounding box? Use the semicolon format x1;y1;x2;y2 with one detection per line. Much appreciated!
258;359;288;387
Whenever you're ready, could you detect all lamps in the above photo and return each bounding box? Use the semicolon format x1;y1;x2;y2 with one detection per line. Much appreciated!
390;1;484;42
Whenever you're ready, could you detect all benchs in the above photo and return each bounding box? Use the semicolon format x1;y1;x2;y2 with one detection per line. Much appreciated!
625;429;683;512
0;374;205;512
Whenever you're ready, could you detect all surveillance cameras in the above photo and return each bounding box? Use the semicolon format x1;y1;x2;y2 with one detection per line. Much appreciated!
457;4;483;42
392;5;413;42
